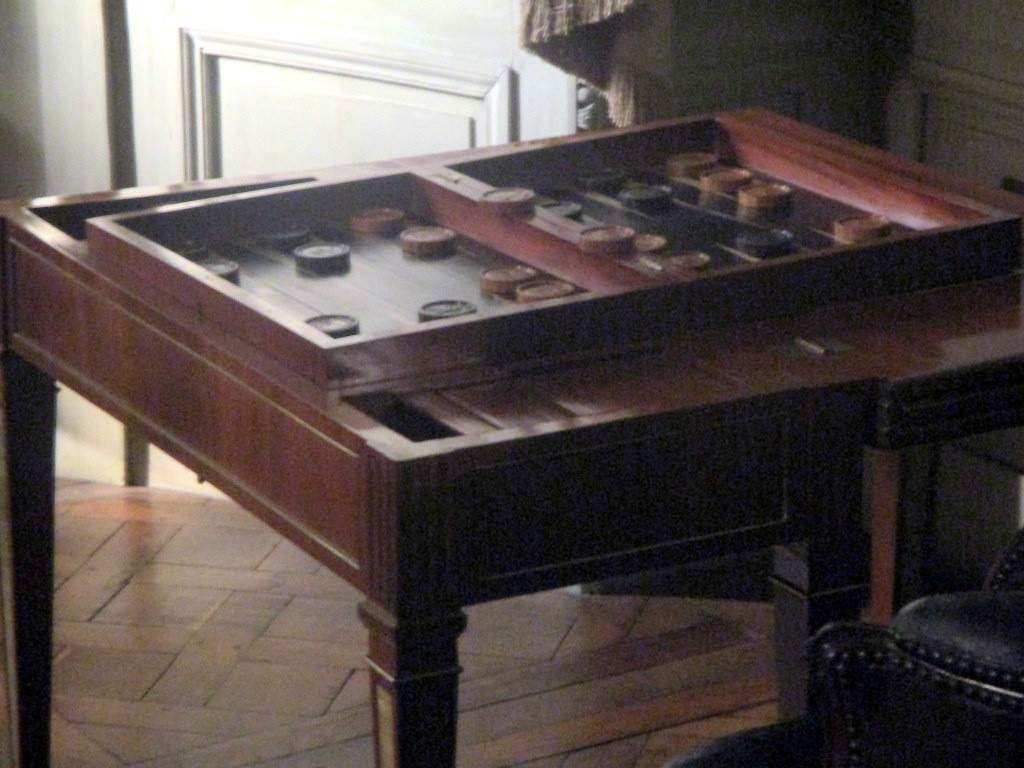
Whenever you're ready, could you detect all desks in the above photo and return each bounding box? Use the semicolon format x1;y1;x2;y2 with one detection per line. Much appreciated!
1;105;1024;766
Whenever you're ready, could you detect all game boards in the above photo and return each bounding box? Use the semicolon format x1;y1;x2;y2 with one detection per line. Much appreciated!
82;111;1023;396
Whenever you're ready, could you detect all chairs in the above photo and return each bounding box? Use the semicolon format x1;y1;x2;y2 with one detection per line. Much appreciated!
662;528;1024;768
